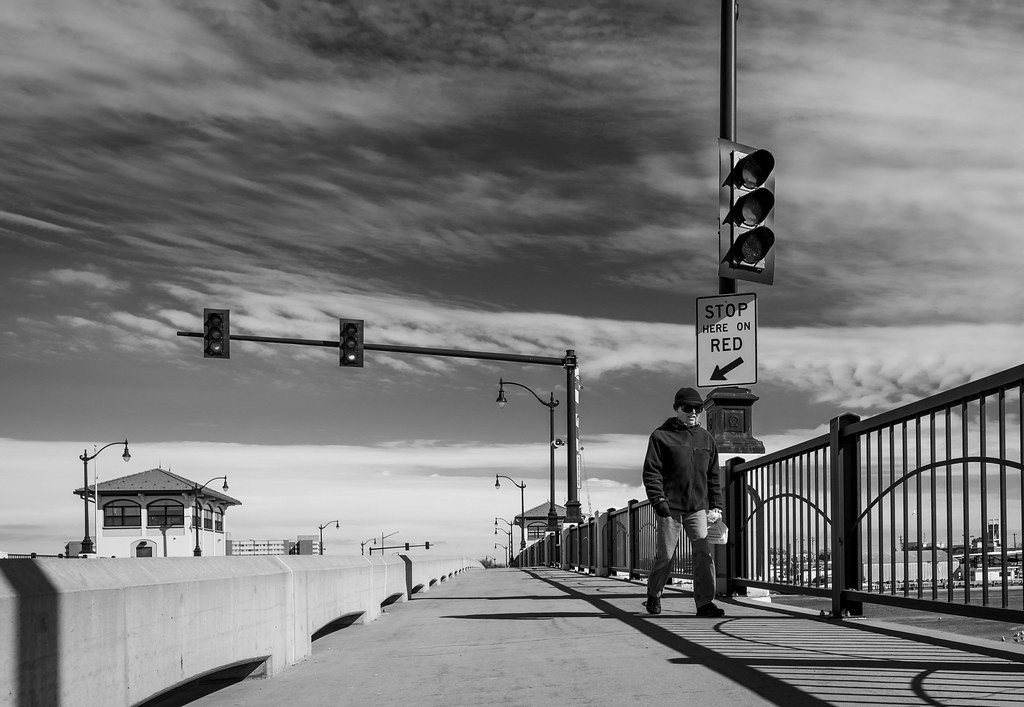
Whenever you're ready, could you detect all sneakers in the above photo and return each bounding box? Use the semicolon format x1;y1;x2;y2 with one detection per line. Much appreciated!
696;602;725;616
647;593;661;614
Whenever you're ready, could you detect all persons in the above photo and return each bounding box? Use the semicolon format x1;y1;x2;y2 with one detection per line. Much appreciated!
641;388;725;617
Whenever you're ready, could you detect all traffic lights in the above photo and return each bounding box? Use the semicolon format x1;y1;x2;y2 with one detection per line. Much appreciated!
204;308;231;359
717;136;776;285
425;542;429;549
340;318;365;367
405;543;409;551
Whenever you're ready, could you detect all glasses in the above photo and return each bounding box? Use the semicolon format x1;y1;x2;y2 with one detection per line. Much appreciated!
681;405;703;413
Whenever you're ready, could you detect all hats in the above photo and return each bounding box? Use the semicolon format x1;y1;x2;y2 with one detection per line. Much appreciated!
673;388;704;412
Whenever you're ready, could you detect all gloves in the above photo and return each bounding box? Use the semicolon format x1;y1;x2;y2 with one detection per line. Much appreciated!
655;502;671;517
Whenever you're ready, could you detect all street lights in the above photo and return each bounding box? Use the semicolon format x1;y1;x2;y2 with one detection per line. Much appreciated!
495;517;514;561
494;542;508;567
494;474;527;549
319;520;340;555
495;527;510;564
194;476;229;555
79;438;131;553
496;377;560;533
361;538;376;556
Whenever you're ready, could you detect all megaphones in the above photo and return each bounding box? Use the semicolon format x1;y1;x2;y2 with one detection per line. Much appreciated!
551;438;563;450
